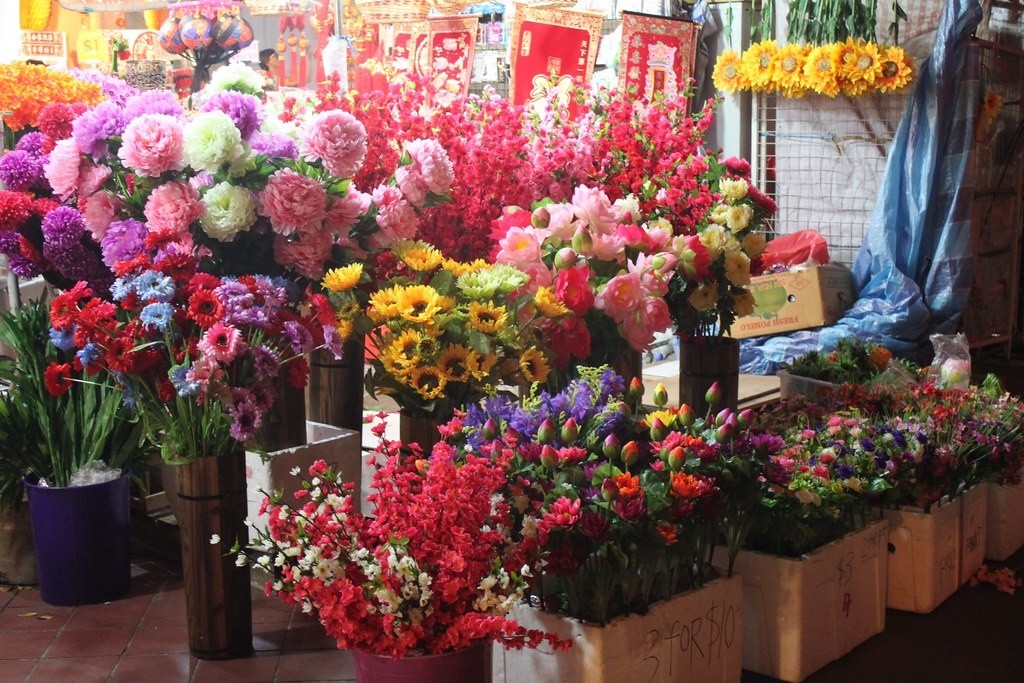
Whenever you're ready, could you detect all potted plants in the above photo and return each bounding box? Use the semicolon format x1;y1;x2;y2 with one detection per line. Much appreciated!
1;297;148;606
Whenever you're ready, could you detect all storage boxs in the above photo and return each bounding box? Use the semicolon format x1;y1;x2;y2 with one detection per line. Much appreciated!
707;516;891;683
885;481;989;614
719;265;854;340
989;479;1024;560
774;369;844;412
492;566;743;682
243;420;361;555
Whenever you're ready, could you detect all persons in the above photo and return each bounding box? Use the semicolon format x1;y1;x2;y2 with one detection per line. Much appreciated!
259;46;311;88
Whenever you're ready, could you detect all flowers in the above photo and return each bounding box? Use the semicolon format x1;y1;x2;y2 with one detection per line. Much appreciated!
0;1;1024;683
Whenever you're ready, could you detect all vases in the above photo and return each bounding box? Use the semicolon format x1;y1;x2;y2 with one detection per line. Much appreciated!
352;645;486;683
253;377;306;451
175;452;252;662
301;333;363;439
679;337;739;420
1;490;39;587
397;412;451;466
